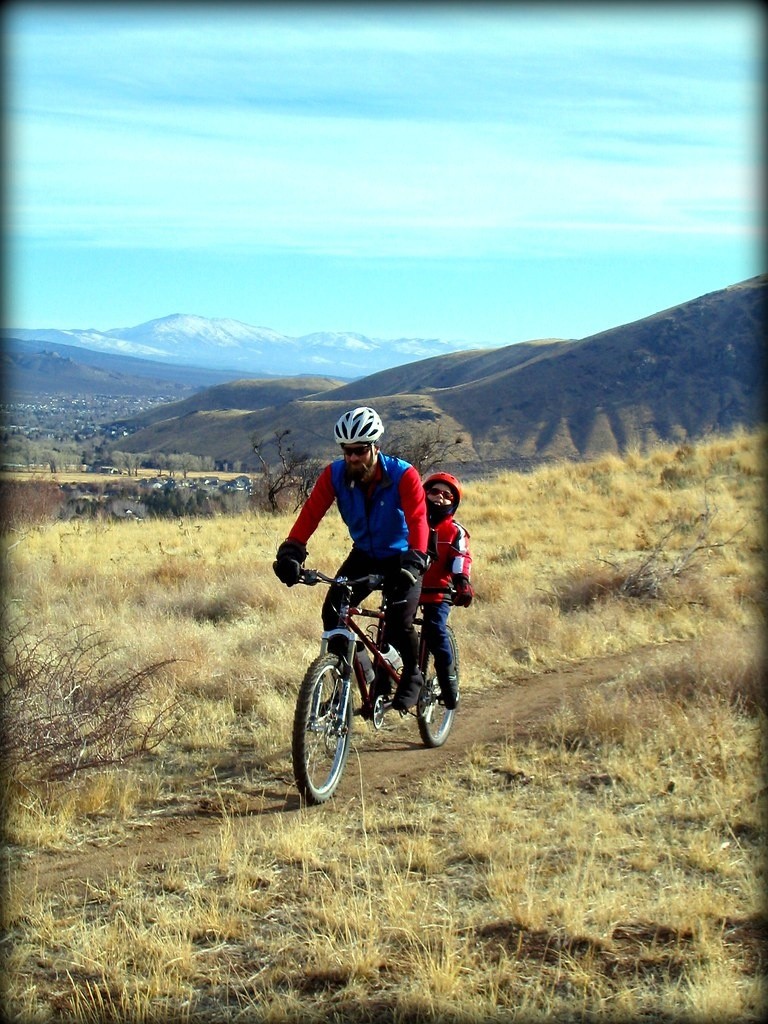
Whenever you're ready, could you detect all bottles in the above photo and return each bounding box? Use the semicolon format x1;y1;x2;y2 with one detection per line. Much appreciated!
379;643;404;670
355;641;375;683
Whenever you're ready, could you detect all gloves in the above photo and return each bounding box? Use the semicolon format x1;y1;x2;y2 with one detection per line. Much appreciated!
382;551;430;602
273;540;307;588
450;573;473;608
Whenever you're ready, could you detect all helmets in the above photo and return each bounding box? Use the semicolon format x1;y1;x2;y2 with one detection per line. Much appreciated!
422;471;462;516
333;407;385;444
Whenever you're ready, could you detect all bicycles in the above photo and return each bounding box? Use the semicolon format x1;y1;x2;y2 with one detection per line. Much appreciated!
271;559;462;807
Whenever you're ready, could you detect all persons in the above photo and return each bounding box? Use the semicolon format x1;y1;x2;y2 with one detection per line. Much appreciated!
414;471;474;711
273;407;432;710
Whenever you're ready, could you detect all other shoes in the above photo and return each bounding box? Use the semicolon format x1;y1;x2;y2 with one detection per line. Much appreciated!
392;670;424;710
434;661;461;710
316;690;346;718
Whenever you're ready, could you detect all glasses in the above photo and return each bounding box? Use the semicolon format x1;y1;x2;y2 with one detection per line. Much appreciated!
426;488;454;501
341;446;370;456
